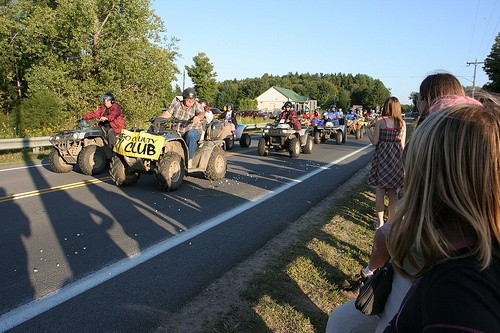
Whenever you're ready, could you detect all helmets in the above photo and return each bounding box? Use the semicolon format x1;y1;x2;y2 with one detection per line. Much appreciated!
182;88;196;99
350;108;354;112
103;93;115;104
330;105;336;109
355;108;361;112
284;101;292;108
198;98;208;106
226;104;233;110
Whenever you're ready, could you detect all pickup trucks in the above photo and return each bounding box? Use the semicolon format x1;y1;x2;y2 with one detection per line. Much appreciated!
235;110;273;119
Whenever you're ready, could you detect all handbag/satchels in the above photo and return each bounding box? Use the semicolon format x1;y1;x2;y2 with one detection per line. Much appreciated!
355;261;394;316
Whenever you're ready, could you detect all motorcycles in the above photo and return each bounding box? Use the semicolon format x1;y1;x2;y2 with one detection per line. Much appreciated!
299;115;380;145
48;114;144;176
109;116;228;192
257;119;315;158
204;117;252;151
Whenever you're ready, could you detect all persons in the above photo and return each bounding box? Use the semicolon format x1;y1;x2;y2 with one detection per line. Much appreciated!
79;92;127;159
277;102;378;145
326;104;500;333
148;88;237;168
415;73;500;128
368;97;406;229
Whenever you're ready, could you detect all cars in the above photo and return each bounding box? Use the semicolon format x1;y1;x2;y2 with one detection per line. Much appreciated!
209;107;224;119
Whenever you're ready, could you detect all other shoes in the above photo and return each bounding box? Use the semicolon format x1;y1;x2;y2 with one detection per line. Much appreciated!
373;223;378;229
188;160;193;168
